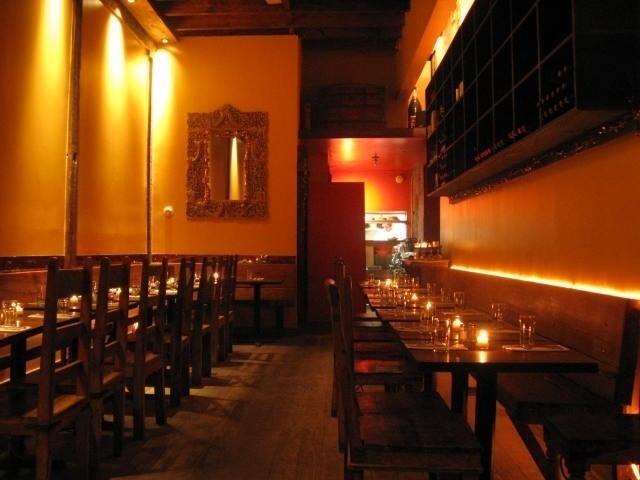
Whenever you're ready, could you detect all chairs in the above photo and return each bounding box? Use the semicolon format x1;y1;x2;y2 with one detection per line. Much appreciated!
351;312;378;318
163;256;197;409
349;319;383;327
218;254;231;362
434;267;640;480
344;272;407;360
323;278;483;480
343;274;452;416
323;278;412;417
103;254;168;445
25;256;131;459
191;256;216;387
192;264;293;339
228;255;238;356
0;257;92;480
352;329;399;343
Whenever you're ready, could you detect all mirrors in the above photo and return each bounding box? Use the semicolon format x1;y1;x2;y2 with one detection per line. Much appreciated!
187;105;268;221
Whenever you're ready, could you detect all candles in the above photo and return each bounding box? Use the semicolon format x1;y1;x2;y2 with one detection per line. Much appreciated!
475;342;490;350
452;326;465;332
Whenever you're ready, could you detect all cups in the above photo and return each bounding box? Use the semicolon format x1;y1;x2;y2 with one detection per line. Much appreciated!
432;317;451;354
488;303;505;329
57;299;70;313
411;276;420;288
403;294;416;321
369;273;375;286
404;274;411;286
453;291;464;311
2;299;18;328
375;280;380;292
440;287;451;303
519;314;537;349
427;282;436;297
386;288;395;305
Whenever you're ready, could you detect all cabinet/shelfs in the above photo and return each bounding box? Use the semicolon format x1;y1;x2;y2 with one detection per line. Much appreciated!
405;259;448;282
425;0;640;200
